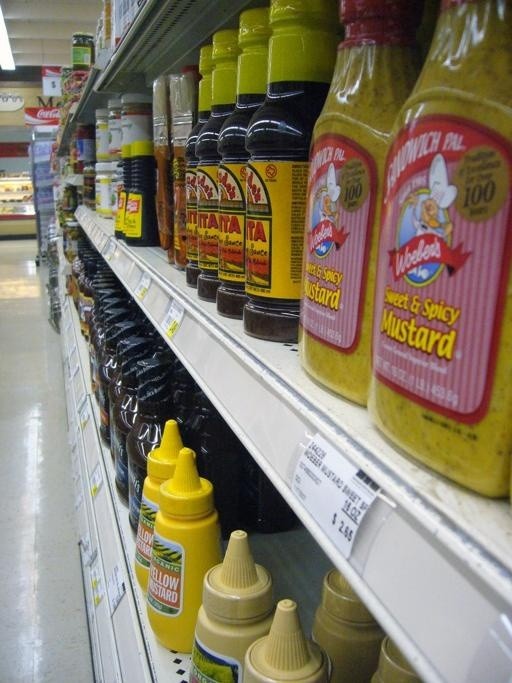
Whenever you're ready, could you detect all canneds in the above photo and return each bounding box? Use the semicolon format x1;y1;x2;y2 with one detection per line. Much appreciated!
72;30;96;70
60;93;154;262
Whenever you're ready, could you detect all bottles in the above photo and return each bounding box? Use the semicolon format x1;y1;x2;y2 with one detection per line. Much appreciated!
70;0;512;683
